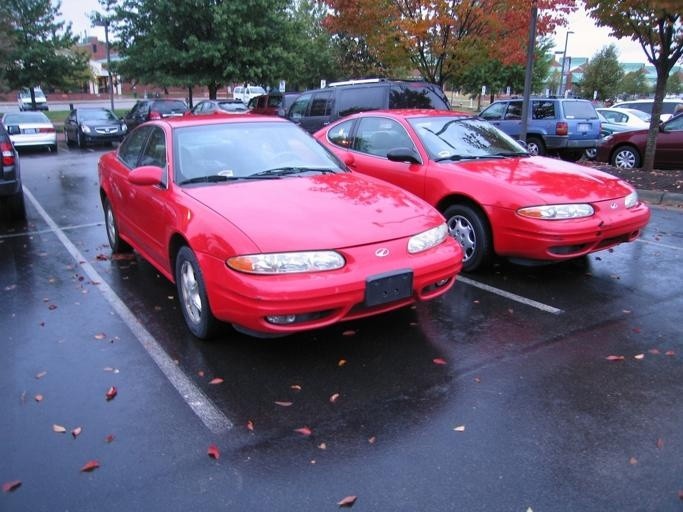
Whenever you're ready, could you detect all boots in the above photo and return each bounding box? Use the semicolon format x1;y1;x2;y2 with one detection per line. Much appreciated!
286;78;445;135
474;96;599;162
610;99;682;123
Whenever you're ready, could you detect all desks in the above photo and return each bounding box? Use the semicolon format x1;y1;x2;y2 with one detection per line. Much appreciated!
95;19;116;112
553;31;575;99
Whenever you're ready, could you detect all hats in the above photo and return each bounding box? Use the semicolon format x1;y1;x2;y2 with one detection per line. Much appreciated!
312;109;651;275
585;108;657;159
0;123;31;226
96;113;466;339
597;110;682;171
